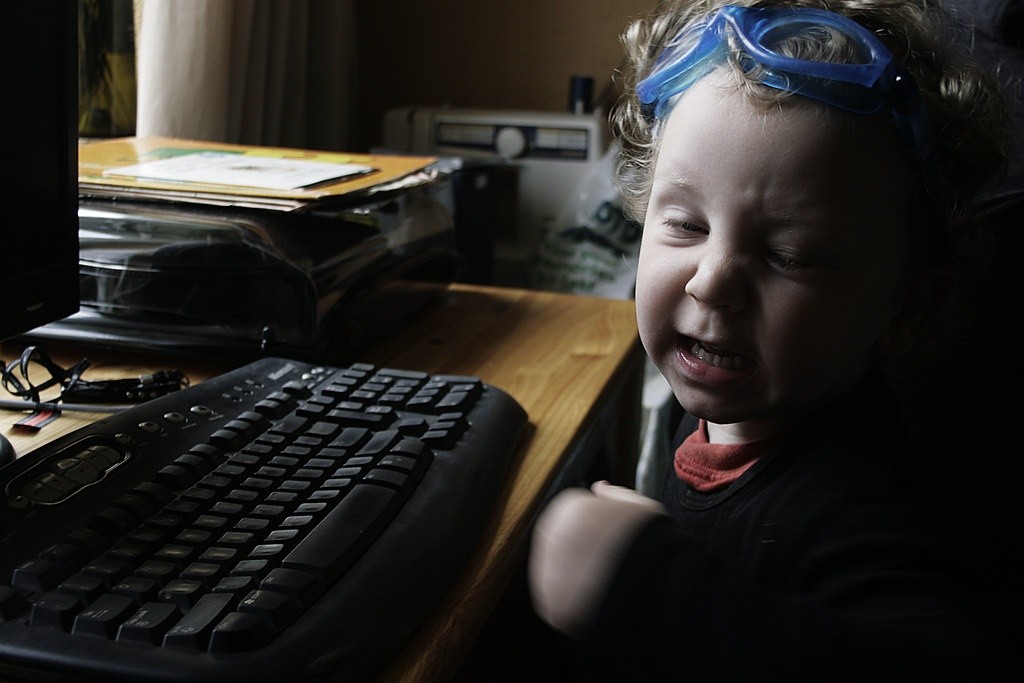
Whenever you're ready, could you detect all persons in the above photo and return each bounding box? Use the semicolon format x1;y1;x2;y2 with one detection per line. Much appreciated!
527;0;1024;683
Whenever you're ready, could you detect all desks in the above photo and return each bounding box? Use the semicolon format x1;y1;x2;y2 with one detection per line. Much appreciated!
0;281;641;683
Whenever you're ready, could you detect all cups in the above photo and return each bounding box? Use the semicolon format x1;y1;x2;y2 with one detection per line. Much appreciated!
567;77;593;116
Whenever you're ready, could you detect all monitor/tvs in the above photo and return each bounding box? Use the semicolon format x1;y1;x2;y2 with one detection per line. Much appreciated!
0;0;82;345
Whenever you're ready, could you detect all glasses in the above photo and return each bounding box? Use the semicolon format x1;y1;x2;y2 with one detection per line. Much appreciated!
634;4;912;187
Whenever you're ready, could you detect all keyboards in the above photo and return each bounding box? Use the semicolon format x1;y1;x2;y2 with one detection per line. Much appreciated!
0;354;528;683
